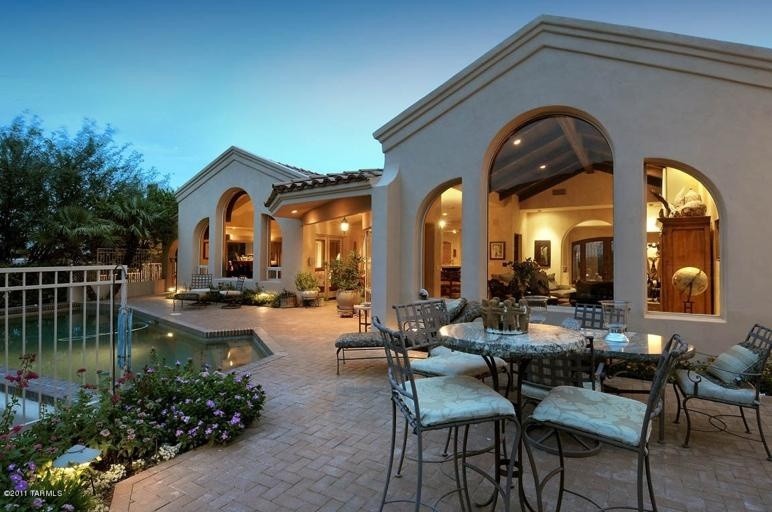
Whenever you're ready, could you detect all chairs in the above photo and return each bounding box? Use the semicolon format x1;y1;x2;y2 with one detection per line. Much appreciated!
598;300;633;332
522;333;688;512
394;300;511;455
218;275;245;310
173;274;213;308
574;303;604;332
370;314;522;512
675;323;772;461
336;298;484;374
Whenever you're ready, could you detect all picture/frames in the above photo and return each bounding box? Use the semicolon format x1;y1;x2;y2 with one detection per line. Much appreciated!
490;242;506;260
534;239;551;266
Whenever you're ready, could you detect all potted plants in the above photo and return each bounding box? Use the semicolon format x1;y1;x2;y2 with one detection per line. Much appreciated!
328;248;367;318
294;271;321;301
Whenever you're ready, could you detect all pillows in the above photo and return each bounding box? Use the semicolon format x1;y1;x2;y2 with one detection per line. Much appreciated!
705;341;766;384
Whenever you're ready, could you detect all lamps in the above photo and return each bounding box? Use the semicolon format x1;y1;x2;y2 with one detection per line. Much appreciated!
341;217;349;239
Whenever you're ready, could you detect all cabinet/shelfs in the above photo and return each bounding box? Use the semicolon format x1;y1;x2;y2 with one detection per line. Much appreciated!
662;216;713;315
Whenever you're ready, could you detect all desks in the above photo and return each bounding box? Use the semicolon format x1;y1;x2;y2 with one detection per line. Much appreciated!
586;328;696;444
436;321;585;458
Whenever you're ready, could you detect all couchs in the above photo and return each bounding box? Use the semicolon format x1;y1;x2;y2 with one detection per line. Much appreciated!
569;280;614;307
546;273;576;298
